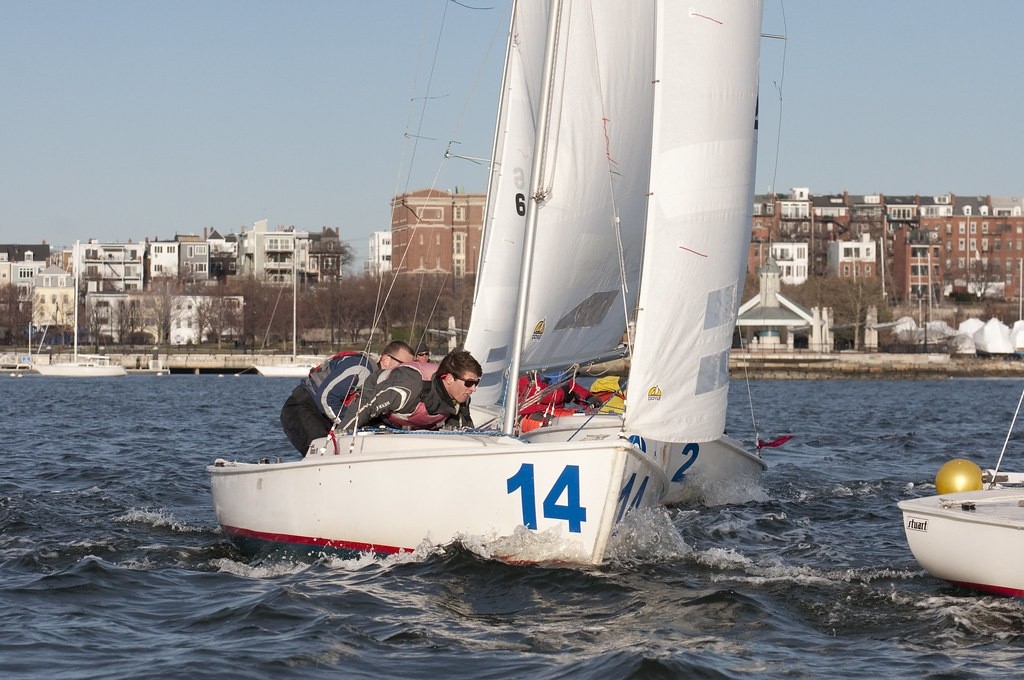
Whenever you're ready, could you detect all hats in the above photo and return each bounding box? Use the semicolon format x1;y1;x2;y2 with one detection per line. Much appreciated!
416;343;430;353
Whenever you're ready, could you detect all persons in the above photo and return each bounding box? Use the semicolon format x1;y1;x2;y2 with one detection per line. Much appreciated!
509;365;629;435
280;340;483;458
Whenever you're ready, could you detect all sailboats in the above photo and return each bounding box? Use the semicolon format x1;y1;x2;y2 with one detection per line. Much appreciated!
27;240;126;376
204;0;768;571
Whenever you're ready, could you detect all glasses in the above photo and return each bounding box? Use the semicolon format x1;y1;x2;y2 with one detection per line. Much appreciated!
416;352;429;356
445;371;480;388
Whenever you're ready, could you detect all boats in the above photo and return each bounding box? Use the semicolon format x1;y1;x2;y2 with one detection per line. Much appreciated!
895;387;1024;600
